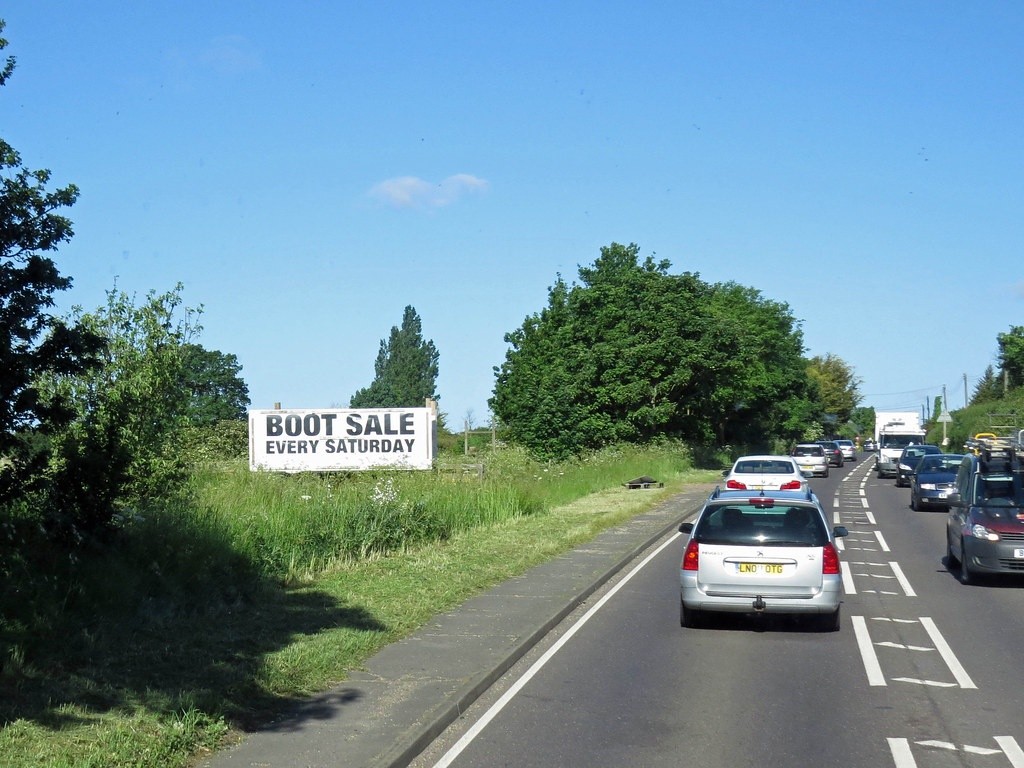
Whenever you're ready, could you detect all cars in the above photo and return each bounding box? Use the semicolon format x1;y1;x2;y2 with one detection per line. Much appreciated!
907;454;964;511
832;439;857;462
721;454;814;514
678;486;849;633
814;440;844;467
791;443;830;478
892;445;942;487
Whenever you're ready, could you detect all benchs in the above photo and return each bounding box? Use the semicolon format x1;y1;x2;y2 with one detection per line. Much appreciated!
744;466;787;473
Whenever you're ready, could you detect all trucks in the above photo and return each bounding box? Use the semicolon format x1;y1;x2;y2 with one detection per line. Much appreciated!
874;412;926;479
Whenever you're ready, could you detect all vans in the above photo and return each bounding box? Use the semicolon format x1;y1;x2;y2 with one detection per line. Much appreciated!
946;449;1024;585
864;439;874;452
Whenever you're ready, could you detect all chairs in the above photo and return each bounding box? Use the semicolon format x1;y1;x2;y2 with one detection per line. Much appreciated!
812;452;818;456
798;452;802;455
908;452;915;457
785;512;808;525
722;508;741;525
